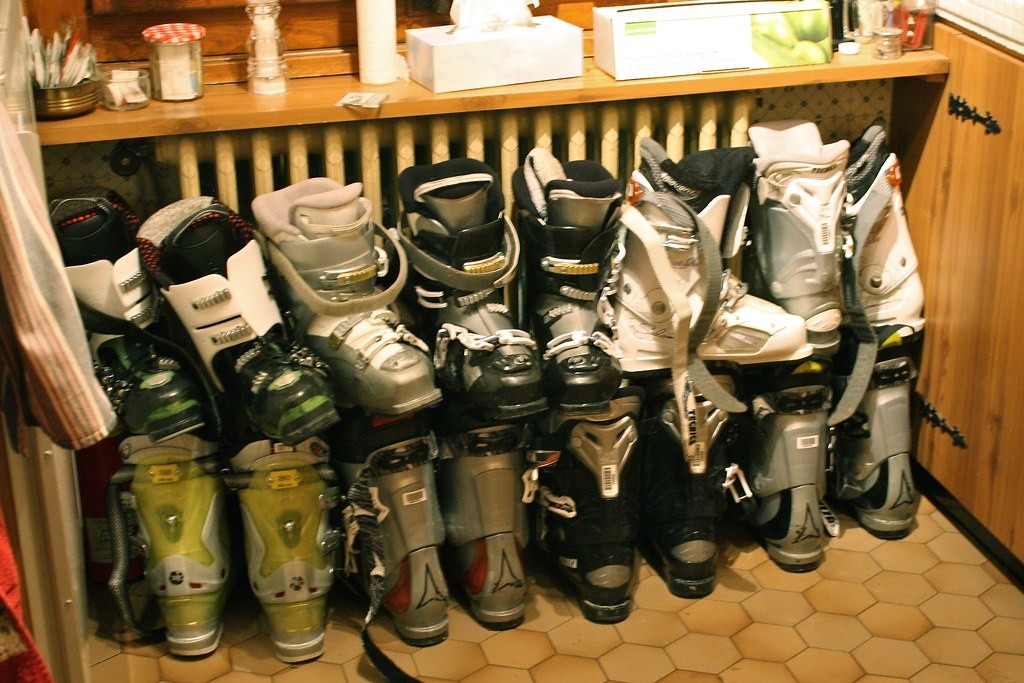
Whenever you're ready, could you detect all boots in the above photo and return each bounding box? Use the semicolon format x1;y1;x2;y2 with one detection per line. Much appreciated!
387;157;551;420
826;120;928;427
330;422;451;683
633;364;742;599
732;119;852;360
826;347;920;541
434;422;532;632
510;147;624;415
45;184;222;445
735;357;840;573
218;434;343;665
250;177;443;417
107;433;235;656
134;194;342;445
517;384;659;623
605;136;814;464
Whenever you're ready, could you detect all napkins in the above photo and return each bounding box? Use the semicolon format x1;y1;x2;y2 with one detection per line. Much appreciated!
592;0;833;83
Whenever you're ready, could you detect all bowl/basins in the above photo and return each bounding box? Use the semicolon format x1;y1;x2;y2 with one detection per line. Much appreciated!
29;78;101;117
838;42;861;55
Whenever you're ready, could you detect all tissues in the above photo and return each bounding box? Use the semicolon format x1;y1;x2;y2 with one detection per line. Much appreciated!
404;0;585;94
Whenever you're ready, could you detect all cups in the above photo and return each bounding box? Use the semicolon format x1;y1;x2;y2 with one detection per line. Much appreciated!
843;0;884;53
143;23;206;100
101;70;152;108
872;28;903;60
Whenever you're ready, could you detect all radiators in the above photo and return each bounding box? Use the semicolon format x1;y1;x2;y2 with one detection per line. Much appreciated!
109;89;756;216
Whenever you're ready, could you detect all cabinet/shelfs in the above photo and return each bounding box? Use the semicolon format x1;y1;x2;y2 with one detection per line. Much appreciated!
886;2;1024;596
